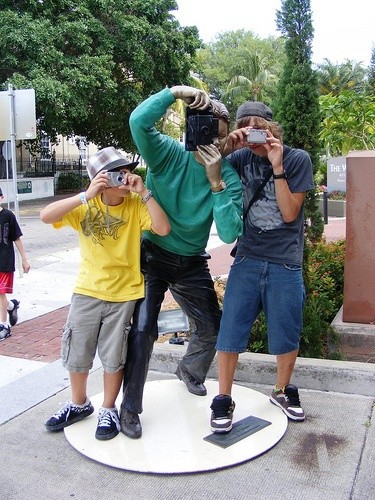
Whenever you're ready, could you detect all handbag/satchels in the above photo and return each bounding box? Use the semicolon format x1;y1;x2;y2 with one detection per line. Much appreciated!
230;238;240;258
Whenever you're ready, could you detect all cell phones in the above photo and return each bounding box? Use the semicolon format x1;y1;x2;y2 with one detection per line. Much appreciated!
185;106;219;151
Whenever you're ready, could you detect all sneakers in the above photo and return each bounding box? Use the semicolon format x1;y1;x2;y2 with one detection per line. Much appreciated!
0;324;11;341
210;394;235;433
46;397;94;431
270;384;306;420
7;299;19;325
95;405;121;441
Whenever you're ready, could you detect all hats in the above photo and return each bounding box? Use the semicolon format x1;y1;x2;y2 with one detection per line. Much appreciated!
85;146;139;181
237;101;272;121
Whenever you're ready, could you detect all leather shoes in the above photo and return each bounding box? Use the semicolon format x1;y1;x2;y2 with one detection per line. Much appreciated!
178;362;207;396
120;403;142;438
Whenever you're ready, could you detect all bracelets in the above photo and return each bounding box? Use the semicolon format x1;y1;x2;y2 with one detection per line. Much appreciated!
79;192;89;203
141;191;153;204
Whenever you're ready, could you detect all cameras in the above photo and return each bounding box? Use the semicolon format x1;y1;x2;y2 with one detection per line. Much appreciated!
104;172;125;187
247;129;268;143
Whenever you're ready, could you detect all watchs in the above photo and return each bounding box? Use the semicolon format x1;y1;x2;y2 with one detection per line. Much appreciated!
212;180;227;193
275;170;288;180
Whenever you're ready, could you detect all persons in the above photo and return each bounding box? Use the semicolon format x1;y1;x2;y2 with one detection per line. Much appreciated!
0;188;30;342
39;146;171;440
117;85;245;439
210;102;315;433
